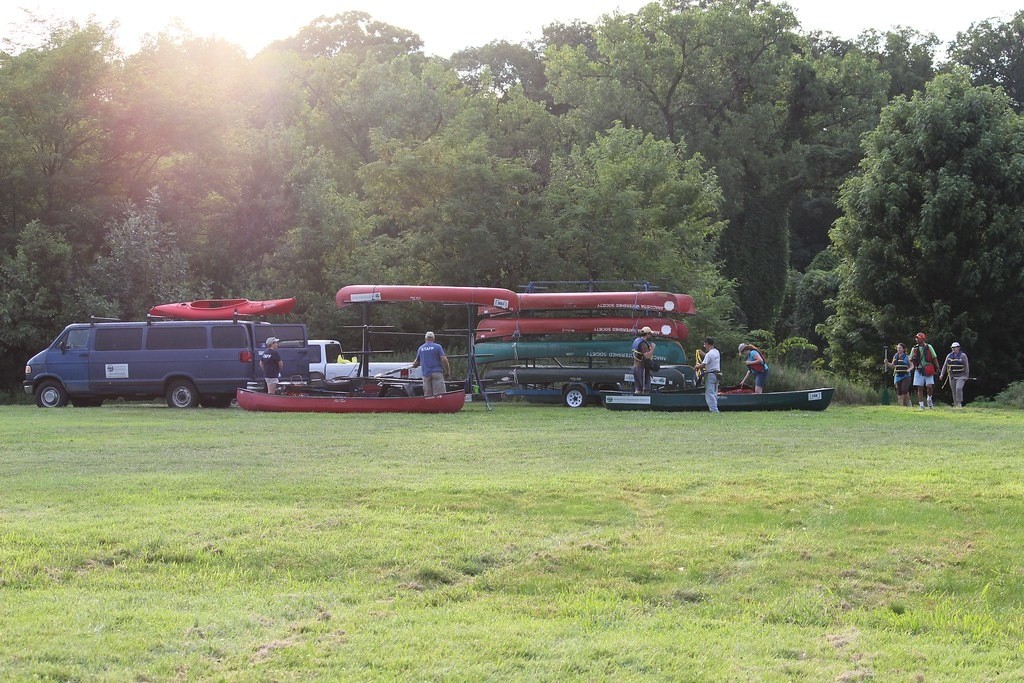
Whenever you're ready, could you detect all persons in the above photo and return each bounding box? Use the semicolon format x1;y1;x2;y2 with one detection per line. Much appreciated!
693;336;721;414
909;332;941;411
258;336;284;394
939;341;969;409
884;343;914;407
631;326;656;394
737;343;769;394
413;331;450;398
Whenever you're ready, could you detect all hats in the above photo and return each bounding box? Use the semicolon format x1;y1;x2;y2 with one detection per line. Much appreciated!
424;331;435;339
641;327;655;335
738;343;746;356
265;336;280;344
950;342;960;348
914;333;926;340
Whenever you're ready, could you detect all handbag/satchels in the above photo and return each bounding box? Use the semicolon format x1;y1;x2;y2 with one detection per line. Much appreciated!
922;364;935;376
643;357;660;371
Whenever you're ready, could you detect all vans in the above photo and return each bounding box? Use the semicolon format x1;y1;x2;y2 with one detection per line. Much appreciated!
22;309;311;407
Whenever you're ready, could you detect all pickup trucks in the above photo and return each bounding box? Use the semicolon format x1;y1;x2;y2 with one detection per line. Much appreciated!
278;340;425;397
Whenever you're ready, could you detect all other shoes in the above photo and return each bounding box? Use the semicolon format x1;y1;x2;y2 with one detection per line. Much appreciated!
919;405;925;410
927;398;934;408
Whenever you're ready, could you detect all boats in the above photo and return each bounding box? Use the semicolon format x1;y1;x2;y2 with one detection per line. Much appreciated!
147;298;296;320
601;386;836;413
336;284;519;315
236;387;466;415
473;292;695;405
